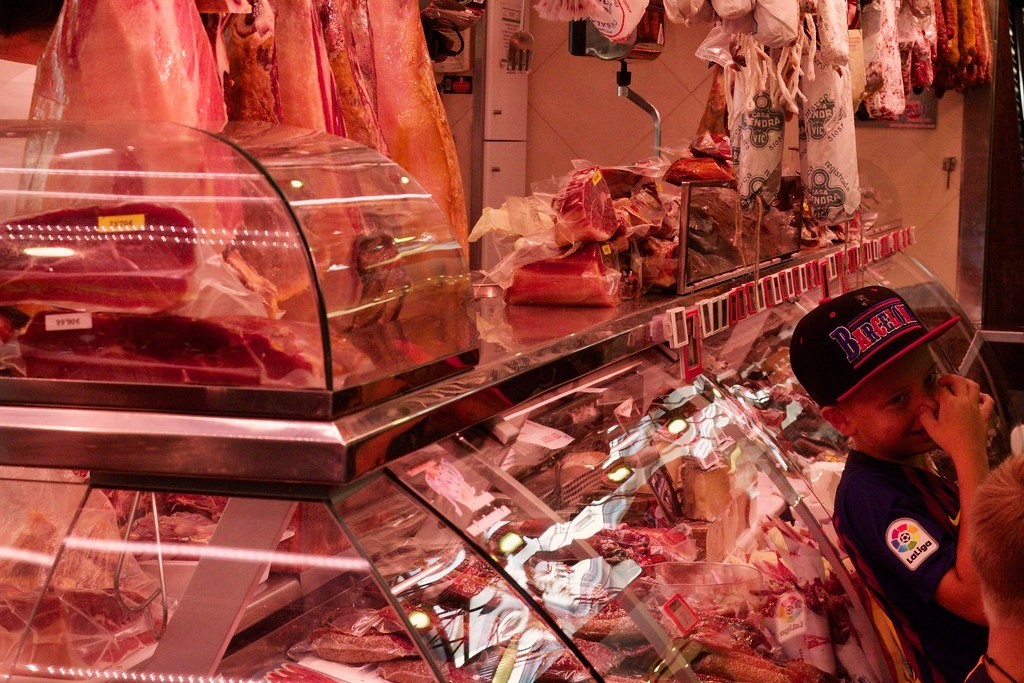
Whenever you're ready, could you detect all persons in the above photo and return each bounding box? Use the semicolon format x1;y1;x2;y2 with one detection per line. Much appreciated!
791;284;1024;683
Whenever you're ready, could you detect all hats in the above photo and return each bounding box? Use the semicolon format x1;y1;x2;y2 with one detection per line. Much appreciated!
789;286;960;406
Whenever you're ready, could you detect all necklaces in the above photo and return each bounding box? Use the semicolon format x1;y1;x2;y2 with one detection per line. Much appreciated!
984;652;1019;683
